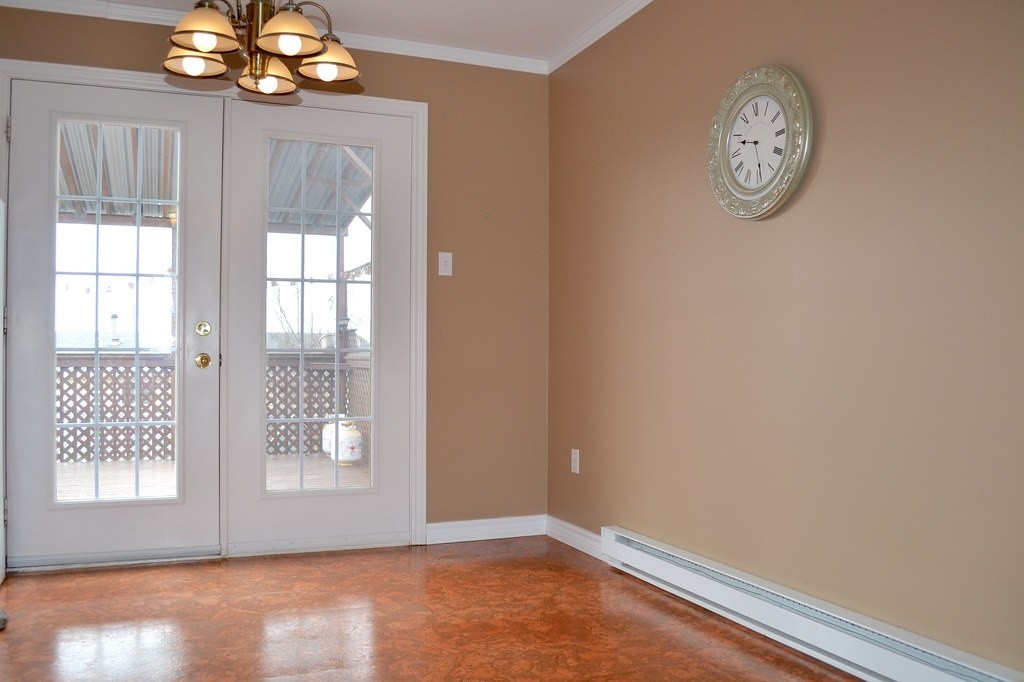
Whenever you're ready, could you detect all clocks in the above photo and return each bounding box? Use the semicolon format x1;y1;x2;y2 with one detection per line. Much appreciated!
707;64;815;221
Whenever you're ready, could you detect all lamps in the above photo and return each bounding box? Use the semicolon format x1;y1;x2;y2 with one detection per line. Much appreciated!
163;0;362;95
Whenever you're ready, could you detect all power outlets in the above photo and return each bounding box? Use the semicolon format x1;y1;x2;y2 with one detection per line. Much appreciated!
438;252;452;276
571;449;580;473
